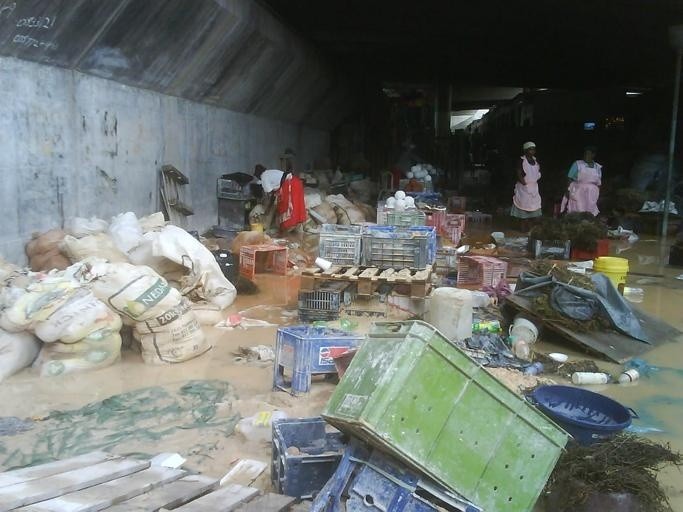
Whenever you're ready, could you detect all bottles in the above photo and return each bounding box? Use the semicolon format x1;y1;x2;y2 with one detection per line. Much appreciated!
571;371;608;383
622;286;645;298
523;362;544;376
311;317;358;332
469;319;502;332
618;367;639;383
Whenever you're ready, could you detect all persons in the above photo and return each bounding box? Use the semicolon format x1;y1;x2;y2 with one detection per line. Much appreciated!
509;139;544;234
252;161;309;236
559;144;604;218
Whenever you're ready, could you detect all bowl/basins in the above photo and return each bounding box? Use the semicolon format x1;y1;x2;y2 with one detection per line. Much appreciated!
548;351;568;363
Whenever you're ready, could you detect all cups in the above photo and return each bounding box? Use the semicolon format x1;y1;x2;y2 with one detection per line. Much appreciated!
313;257;331;274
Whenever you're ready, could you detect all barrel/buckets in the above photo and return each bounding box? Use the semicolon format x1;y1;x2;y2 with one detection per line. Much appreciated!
251;222;264;232
592;255;630;296
429;287;473;343
399;162;444;191
508;312;540;350
386;190;416;212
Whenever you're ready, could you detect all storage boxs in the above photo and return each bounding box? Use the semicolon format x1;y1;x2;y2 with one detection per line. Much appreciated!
320;320;574;512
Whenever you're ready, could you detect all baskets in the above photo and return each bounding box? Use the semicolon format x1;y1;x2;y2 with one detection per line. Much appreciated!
320;178;465;270
297;283;352;324
457;255;509;290
528;236;571;259
239;241;288;281
467;211;494;229
